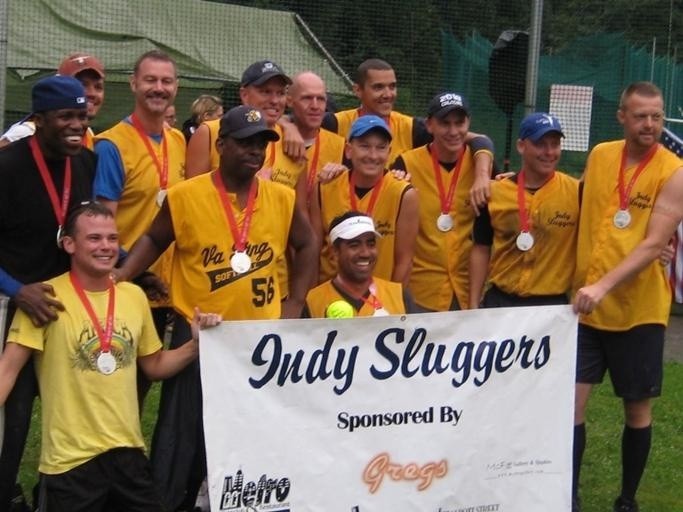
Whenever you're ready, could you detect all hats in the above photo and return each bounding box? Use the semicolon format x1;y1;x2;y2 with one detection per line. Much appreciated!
330;216;382;244
518;112;565;143
59;54;104;80
348;115;392;142
428;93;469;119
241;62;293;87
218;104;280;141
20;73;86;126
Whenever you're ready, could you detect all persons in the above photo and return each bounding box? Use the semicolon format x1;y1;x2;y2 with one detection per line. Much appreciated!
389;91;499;314
184;59;309;267
1;49;227;421
468;111;675;310
493;81;683;511
301;210;414;318
273;56;493;218
284;70;353;210
0;203;223;511
307;113;419;314
0;73;99;511
106;103;320;510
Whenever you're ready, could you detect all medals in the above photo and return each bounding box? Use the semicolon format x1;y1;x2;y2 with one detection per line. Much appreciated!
229;245;252;274
515;229;534;252
372;305;388;318
613;206;632;229
436;211;454;232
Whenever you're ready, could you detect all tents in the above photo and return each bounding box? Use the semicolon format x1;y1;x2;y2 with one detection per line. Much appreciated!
1;1;361;137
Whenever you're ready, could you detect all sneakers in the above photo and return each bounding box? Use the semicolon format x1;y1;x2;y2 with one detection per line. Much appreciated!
194;482;211;512
